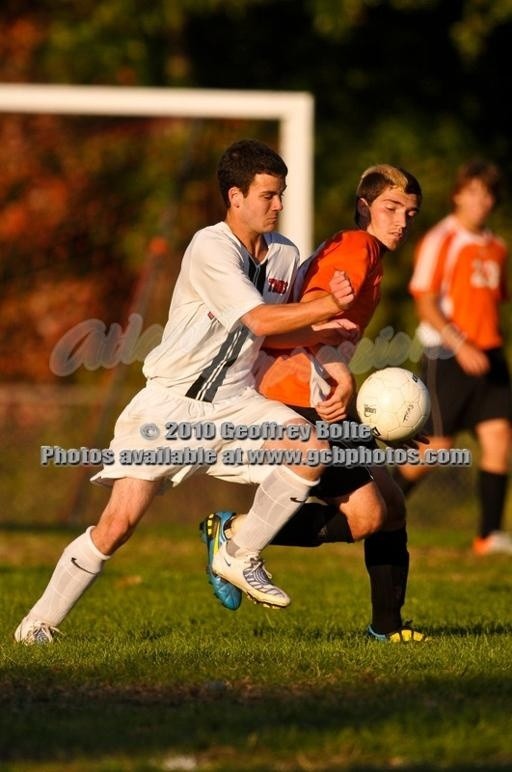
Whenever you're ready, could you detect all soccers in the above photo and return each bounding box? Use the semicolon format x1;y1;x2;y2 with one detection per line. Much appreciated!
356;368;430;443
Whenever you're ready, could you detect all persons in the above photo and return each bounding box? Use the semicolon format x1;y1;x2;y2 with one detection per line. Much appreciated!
198;163;432;644
15;142;363;647
390;159;512;558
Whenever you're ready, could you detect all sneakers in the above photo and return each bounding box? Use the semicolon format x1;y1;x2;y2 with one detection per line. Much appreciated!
211;541;293;610
471;530;512;558
12;615;67;645
199;511;242;610
365;621;433;643
